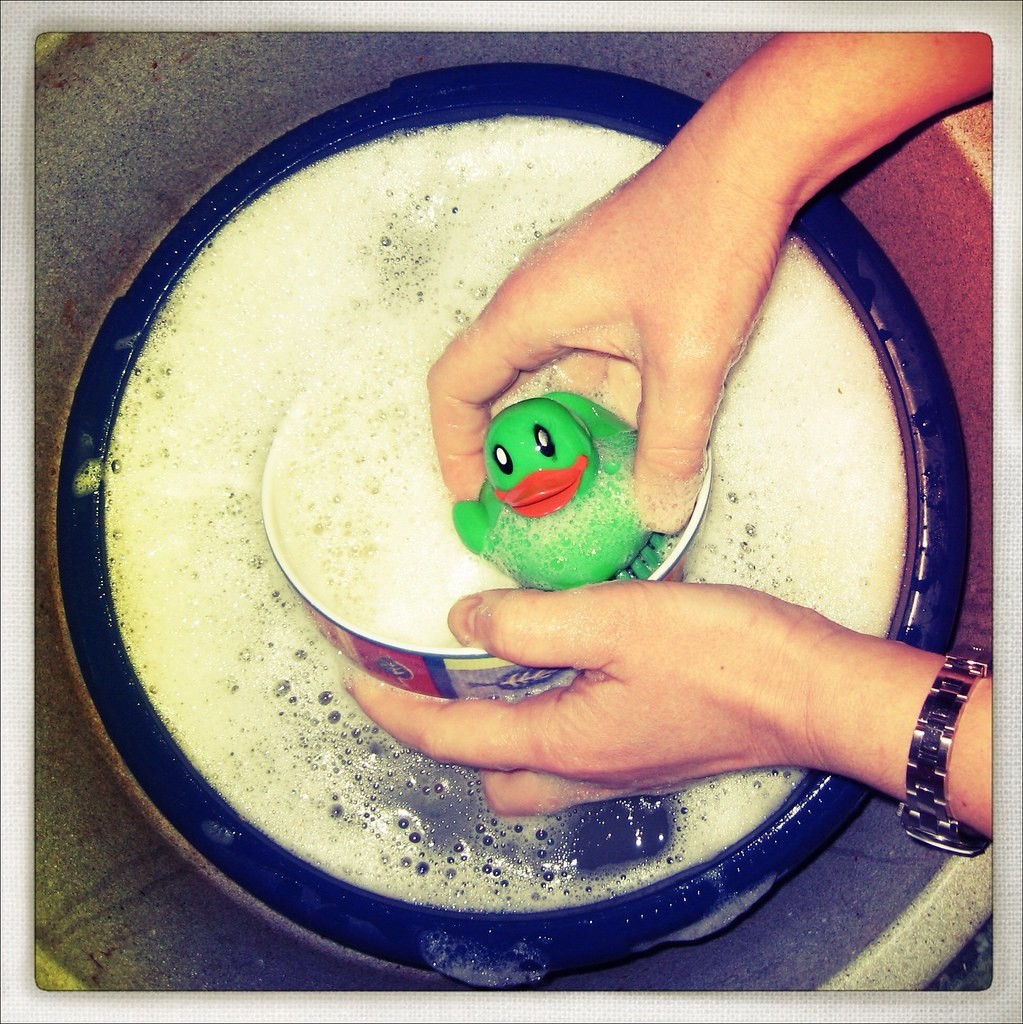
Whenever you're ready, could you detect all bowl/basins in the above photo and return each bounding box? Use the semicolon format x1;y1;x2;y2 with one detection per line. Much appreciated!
259;358;713;708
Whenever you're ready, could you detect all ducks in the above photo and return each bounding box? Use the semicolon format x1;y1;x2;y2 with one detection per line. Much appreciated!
452;390;654;591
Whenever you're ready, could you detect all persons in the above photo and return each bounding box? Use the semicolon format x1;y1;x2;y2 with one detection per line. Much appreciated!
338;32;992;841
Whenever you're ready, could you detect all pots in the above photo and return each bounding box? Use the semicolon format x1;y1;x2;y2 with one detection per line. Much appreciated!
46;61;969;987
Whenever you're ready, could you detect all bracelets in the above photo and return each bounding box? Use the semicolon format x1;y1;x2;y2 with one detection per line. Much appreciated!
896;639;992;858
